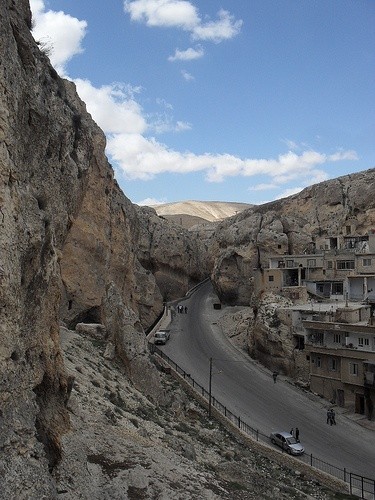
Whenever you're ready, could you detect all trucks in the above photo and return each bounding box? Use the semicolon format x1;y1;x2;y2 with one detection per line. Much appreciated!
153;329;171;345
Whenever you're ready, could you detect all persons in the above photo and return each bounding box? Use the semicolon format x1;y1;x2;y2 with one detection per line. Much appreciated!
178;305;183;314
273;371;278;383
290;428;294;435
327;409;336;426
185;306;187;314
296;428;299;440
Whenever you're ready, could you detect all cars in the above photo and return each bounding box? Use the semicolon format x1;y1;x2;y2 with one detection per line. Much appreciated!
270;431;305;456
